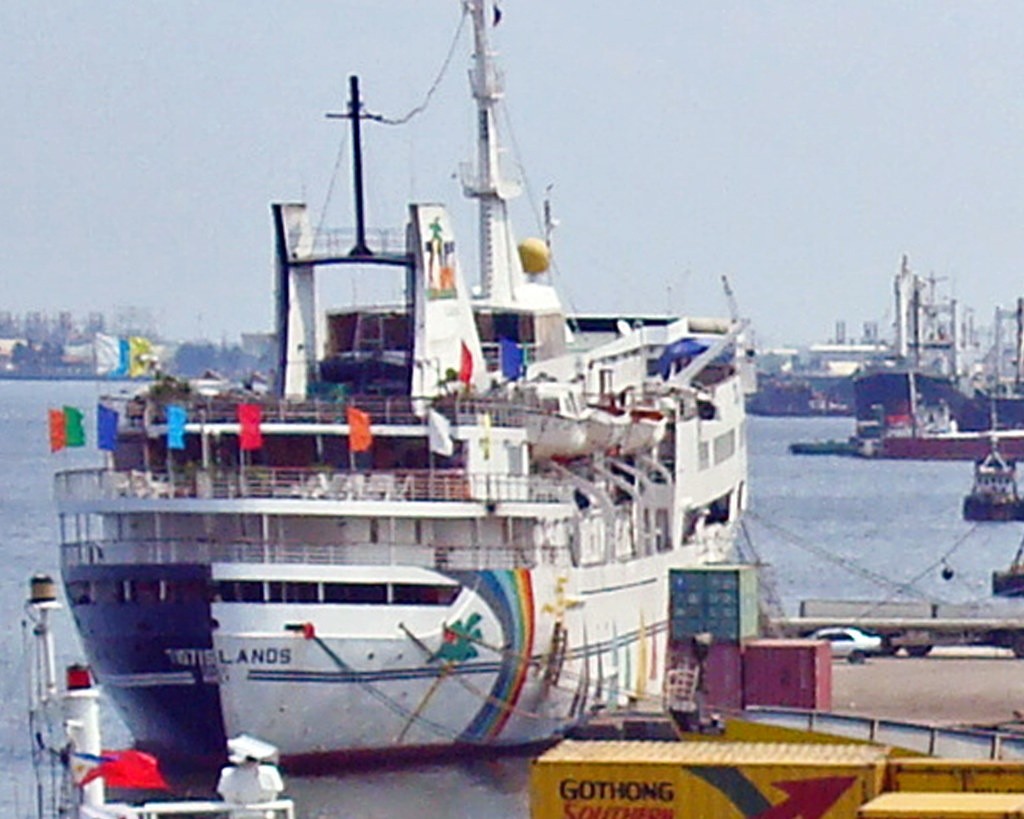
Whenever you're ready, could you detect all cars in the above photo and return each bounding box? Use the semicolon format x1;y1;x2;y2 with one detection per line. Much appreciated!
807;627;882;666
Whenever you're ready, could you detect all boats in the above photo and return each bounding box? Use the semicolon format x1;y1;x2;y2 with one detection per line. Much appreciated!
585;405;632;450
963;435;1024;522
991;564;1023;598
507;381;593;459
55;1;752;762
851;354;1024;432
622;409;670;454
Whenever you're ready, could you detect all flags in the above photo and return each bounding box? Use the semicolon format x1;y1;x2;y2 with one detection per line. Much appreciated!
49;408;65;451
344;404;373;453
63;404;86;447
499;336;522;379
461;341;472;381
167;404;188;449
235;402;265;451
91;331;155;378
428;405;454;457
96;403;119;452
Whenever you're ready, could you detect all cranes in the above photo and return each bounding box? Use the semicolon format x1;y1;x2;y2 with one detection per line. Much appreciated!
722;275;740;317
